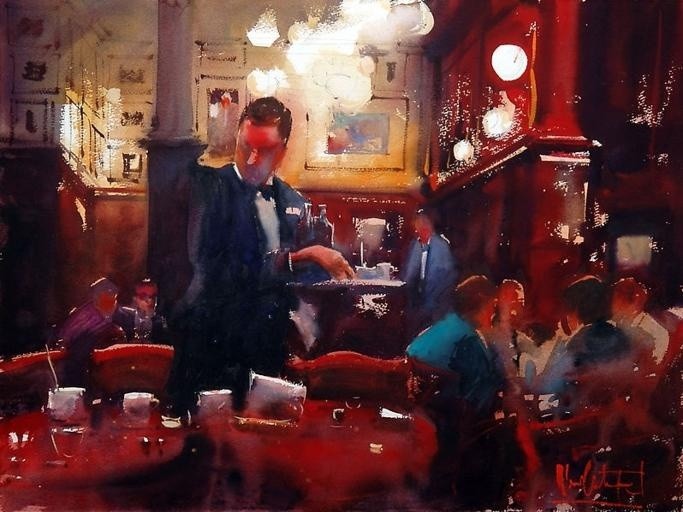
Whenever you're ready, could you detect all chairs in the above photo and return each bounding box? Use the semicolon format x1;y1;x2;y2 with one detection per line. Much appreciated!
0;296;682;512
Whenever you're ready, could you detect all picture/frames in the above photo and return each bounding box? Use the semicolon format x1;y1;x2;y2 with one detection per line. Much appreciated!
1;0;412;185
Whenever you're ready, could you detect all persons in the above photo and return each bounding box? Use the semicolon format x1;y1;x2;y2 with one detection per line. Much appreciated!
157;95;357;423
41;202;682;511
